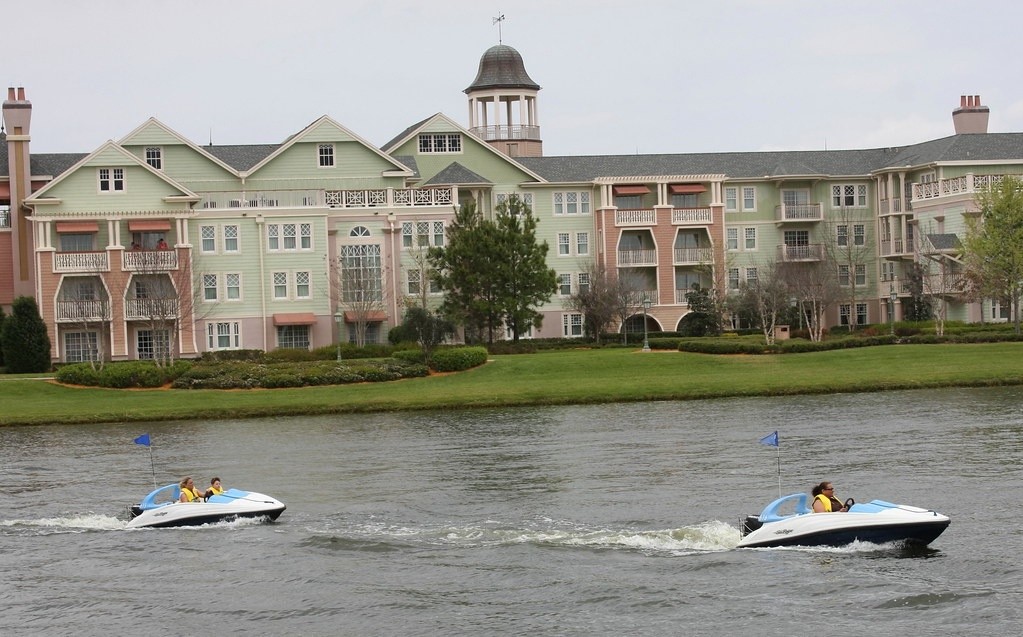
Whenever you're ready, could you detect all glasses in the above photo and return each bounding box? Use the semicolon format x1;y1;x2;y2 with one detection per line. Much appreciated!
825;487;834;490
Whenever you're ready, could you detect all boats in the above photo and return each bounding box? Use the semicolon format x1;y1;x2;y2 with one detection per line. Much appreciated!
737;430;951;549
126;431;286;529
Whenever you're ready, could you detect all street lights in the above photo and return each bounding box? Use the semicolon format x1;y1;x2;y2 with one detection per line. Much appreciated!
888;283;897;335
334;303;342;364
641;289;653;352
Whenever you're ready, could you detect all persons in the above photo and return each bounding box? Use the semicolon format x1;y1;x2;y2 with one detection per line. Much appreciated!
811;481;850;514
179;476;210;504
207;478;226;495
